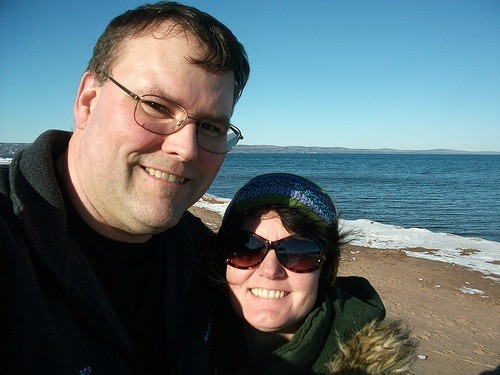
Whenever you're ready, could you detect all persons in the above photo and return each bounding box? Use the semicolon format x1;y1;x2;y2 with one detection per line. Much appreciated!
178;173;422;375
0;0;251;375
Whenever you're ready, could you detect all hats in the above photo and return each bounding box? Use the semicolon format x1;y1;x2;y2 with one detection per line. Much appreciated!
222;173;338;243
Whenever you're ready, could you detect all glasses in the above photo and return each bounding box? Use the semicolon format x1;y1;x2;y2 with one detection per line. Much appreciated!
222;229;325;273
104;73;244;154
